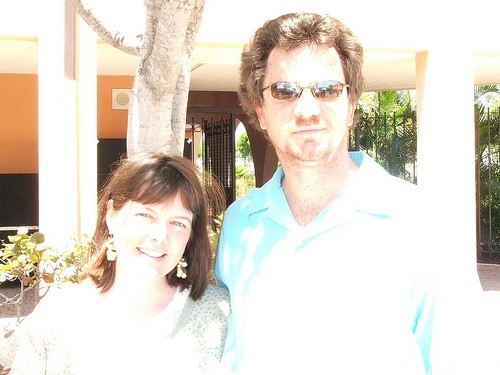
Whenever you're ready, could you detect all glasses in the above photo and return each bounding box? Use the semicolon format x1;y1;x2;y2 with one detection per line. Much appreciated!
261;80;351;102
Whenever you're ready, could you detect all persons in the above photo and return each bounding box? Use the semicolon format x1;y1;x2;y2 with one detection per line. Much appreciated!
212;13;499;375
5;149;233;375
